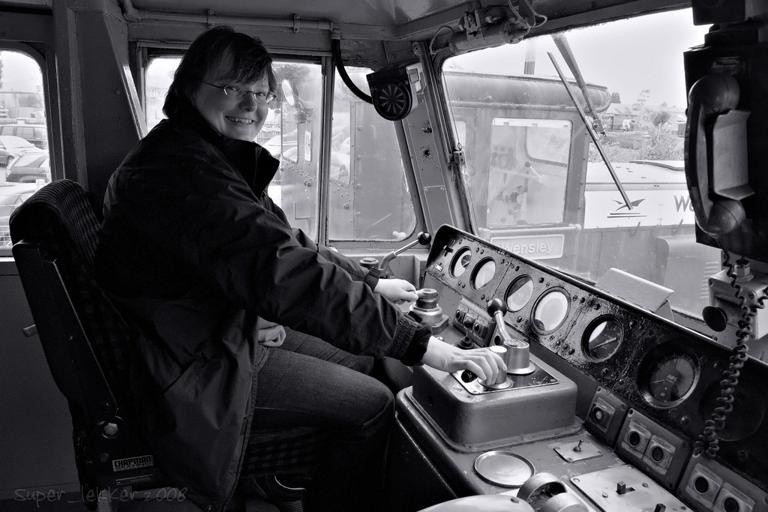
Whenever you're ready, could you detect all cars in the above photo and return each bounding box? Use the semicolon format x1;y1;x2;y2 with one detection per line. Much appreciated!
0;104;52;242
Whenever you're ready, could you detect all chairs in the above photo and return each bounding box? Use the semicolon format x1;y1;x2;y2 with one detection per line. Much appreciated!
6;179;371;511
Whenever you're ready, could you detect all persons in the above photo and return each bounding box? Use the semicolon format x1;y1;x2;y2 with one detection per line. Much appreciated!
87;24;509;512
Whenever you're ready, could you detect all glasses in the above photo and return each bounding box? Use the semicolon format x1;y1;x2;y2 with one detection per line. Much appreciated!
197;80;277;105
